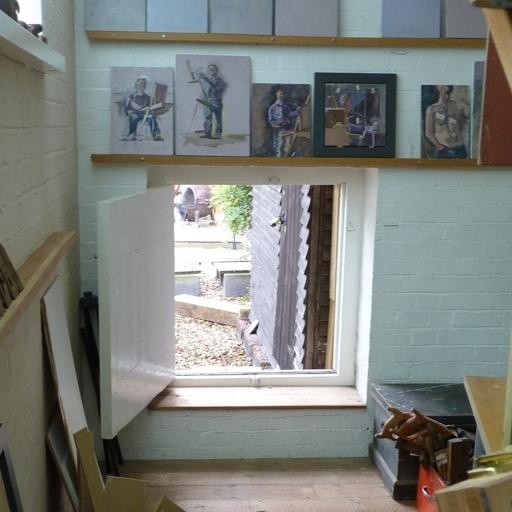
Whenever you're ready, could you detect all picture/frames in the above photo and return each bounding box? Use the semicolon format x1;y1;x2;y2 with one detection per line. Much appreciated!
314;71;397;158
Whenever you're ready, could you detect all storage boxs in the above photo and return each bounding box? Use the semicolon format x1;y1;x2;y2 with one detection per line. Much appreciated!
415;462;449;512
370;383;477;501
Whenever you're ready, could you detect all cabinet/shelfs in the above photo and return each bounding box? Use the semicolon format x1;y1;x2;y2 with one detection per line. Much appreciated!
85;30;486;167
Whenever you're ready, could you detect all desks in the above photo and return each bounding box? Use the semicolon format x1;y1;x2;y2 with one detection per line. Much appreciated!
463;376;507;469
